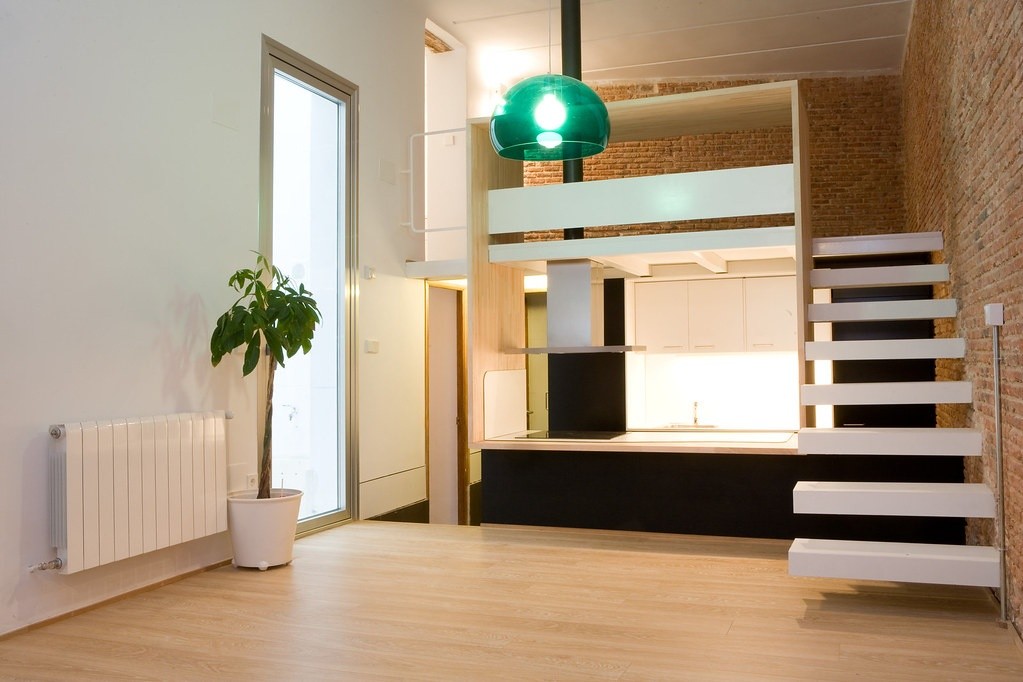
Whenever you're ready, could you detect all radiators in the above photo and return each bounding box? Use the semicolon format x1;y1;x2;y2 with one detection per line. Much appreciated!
27;409;235;576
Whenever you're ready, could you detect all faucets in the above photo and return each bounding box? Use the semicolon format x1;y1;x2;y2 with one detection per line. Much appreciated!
693;402;698;424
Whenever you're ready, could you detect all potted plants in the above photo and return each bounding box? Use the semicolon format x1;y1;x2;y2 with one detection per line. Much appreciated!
210;249;325;572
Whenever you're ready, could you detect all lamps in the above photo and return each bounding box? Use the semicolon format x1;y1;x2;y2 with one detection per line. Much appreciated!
489;0;611;162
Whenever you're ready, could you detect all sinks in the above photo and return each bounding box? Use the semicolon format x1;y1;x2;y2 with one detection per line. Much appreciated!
664;424;717;429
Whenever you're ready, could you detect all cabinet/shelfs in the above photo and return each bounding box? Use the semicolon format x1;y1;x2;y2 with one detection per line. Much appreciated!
467;78;815;456
632;275;799;353
